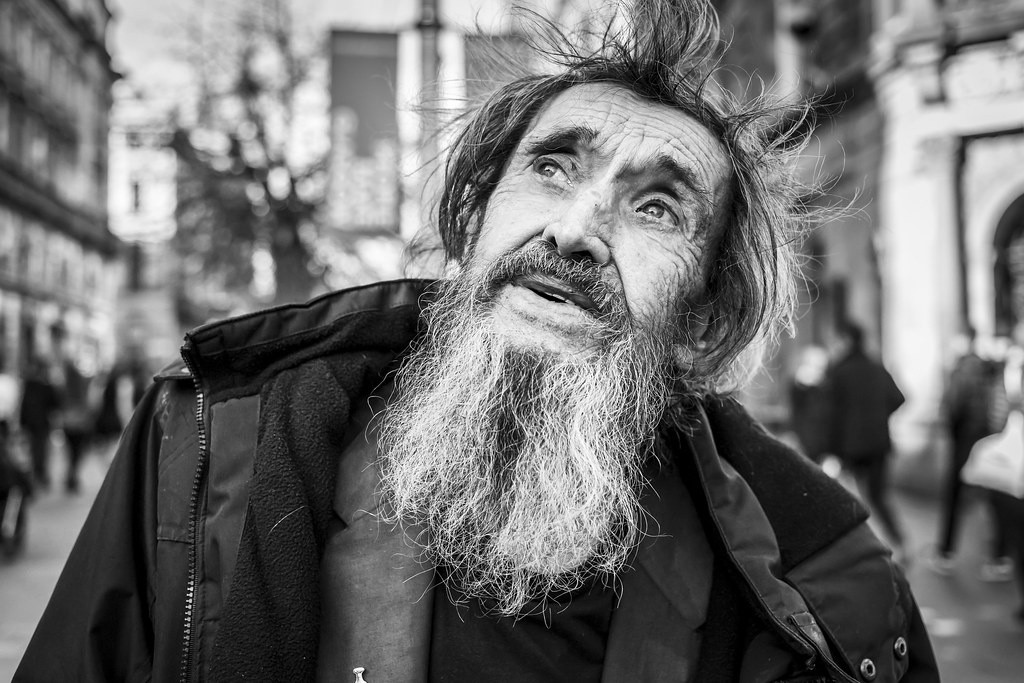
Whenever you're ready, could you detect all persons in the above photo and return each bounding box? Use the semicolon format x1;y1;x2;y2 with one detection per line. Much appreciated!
13;0;945;683
0;312;1024;636
